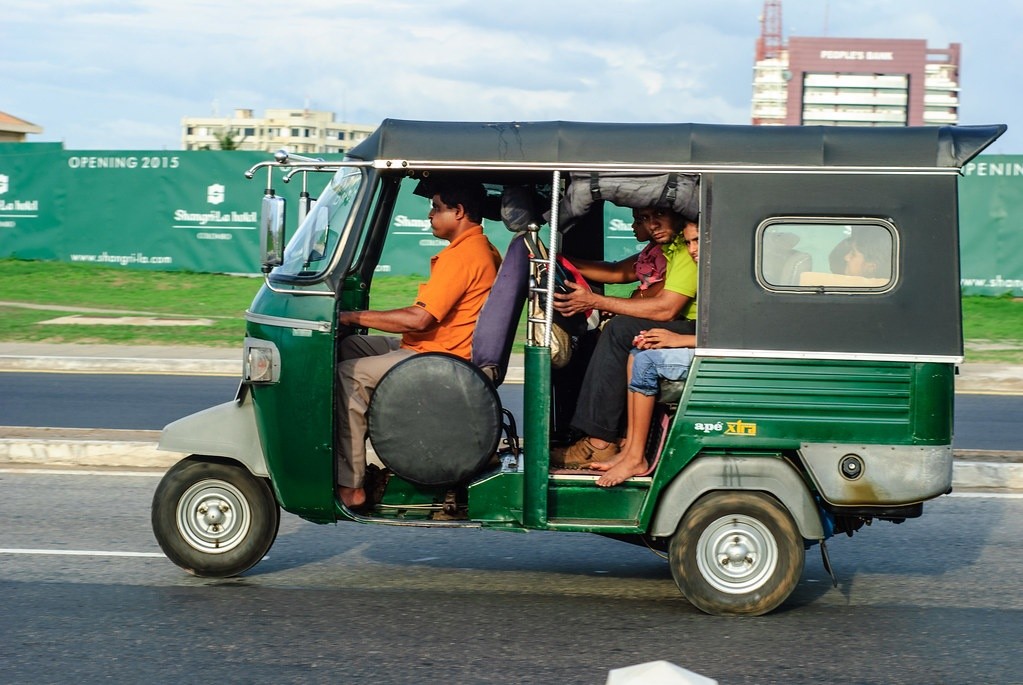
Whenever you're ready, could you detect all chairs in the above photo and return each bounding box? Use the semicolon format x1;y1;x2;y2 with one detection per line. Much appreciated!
365;230;531;516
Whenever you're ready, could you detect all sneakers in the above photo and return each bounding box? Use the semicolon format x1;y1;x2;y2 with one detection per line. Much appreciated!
549;436;620;471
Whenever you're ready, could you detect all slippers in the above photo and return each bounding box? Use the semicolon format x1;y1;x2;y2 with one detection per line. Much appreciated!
348;503;369;515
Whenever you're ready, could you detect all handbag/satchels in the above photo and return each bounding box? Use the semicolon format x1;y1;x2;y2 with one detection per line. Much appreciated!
525;232;601;366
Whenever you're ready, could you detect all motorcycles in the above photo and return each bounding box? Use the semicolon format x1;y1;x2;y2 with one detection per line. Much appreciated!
152;121;1011;620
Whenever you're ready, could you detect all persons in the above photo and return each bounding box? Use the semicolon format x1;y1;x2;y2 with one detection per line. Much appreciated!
335;172;501;508
550;204;893;485
